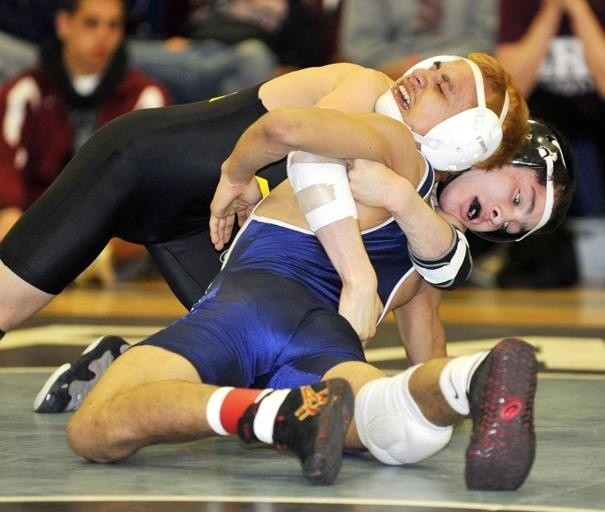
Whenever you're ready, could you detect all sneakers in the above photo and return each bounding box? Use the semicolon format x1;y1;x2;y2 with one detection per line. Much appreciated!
464;338;537;491
273;379;353;485
33;334;129;413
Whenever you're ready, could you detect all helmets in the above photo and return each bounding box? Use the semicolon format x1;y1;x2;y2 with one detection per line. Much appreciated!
435;117;569;246
372;54;510;173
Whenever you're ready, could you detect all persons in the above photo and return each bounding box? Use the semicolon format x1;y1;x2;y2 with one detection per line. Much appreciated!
0;60;579;415
1;1;605;293
64;49;539;491
